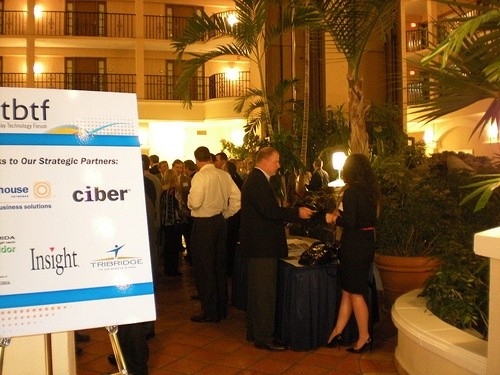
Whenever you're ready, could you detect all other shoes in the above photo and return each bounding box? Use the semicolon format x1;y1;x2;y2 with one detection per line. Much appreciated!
164;271;182;276
75;334;89;341
191;313;219;323
76;347;82;354
217;313;225;319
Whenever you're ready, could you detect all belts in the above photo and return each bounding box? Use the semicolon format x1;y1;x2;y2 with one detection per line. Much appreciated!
354;227;375;230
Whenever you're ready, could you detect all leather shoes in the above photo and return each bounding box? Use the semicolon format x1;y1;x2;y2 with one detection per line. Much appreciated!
108;355;116;365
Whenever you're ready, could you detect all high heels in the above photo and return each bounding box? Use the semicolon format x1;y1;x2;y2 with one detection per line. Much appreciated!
324;335;342;350
347;336;373;354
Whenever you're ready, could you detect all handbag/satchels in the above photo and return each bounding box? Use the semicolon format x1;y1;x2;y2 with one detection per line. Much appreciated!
298;241;338;268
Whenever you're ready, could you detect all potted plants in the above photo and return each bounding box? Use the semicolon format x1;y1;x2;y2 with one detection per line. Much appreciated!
377;192;460;315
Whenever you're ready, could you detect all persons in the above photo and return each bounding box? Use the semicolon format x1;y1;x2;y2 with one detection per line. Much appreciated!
239;147;314;352
143;155;329;307
325;153;379;355
187;147;242;323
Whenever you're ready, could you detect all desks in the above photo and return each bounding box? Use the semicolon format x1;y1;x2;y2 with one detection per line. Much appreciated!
231;235;380;352
